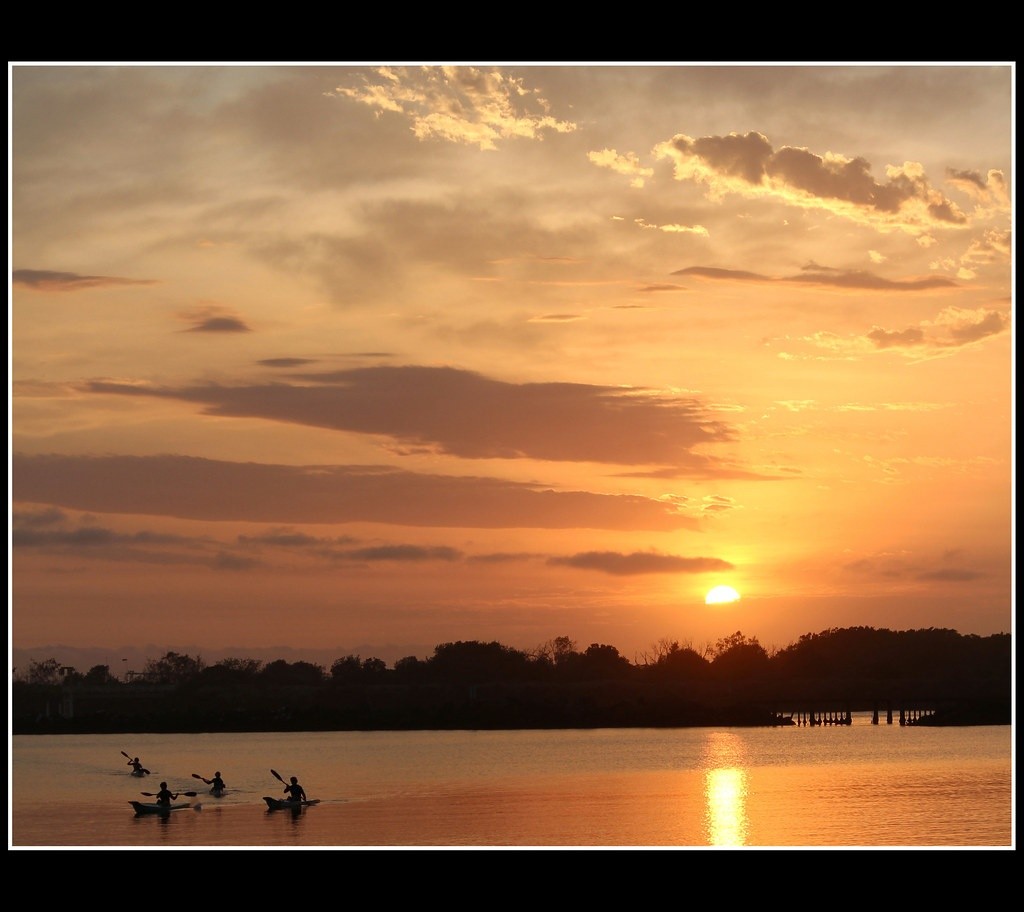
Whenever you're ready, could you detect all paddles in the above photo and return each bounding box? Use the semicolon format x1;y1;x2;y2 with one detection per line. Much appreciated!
192;773;226;788
270;769;308;805
121;751;151;774
140;791;198;797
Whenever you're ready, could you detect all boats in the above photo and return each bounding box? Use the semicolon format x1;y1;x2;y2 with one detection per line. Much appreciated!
262;796;320;811
128;800;191;815
131;770;144;777
210;789;223;798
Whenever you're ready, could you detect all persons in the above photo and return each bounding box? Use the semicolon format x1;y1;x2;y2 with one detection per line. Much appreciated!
156;782;179;805
283;777;306;802
127;757;142;773
203;771;223;793
